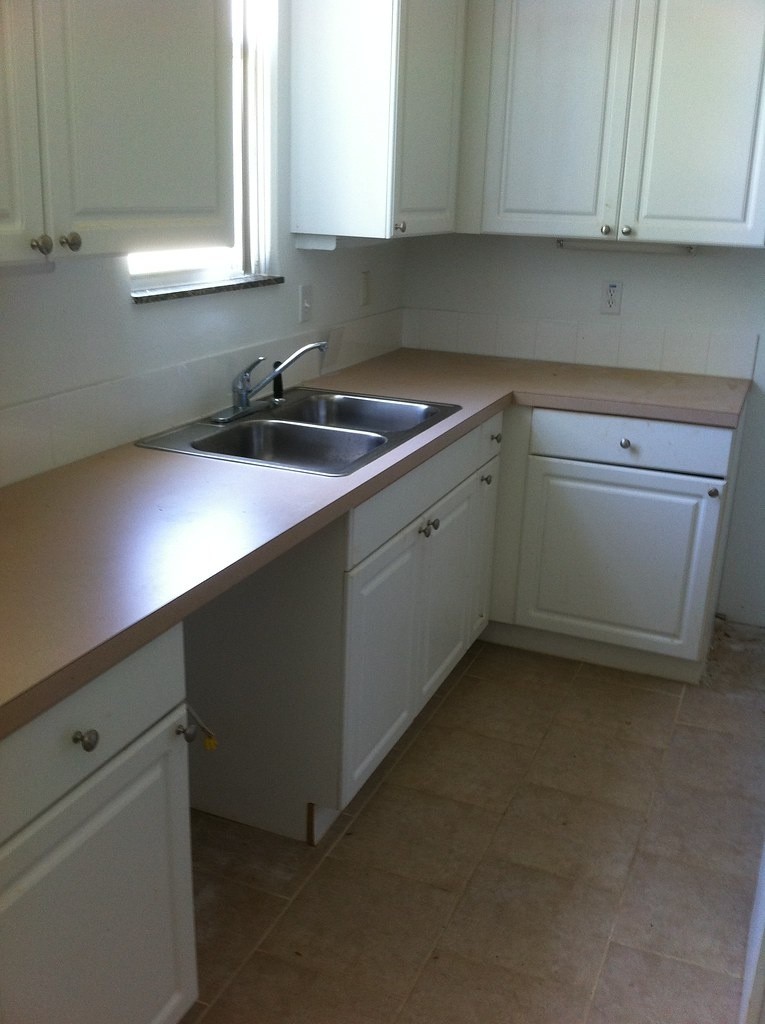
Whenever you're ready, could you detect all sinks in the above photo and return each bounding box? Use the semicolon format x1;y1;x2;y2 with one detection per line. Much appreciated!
268;387;462;434
134;419;388;476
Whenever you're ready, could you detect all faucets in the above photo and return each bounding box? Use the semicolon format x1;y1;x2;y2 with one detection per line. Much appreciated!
232;342;328;408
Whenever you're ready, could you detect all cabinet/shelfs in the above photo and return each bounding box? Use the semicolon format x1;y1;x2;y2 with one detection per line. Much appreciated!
288;0;467;240
0;0;234;263
182;410;505;848
0;622;200;1024
455;0;765;247
515;408;753;688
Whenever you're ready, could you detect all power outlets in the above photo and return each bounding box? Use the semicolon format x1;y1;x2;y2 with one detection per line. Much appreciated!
360;270;370;307
599;280;623;316
299;284;313;324
